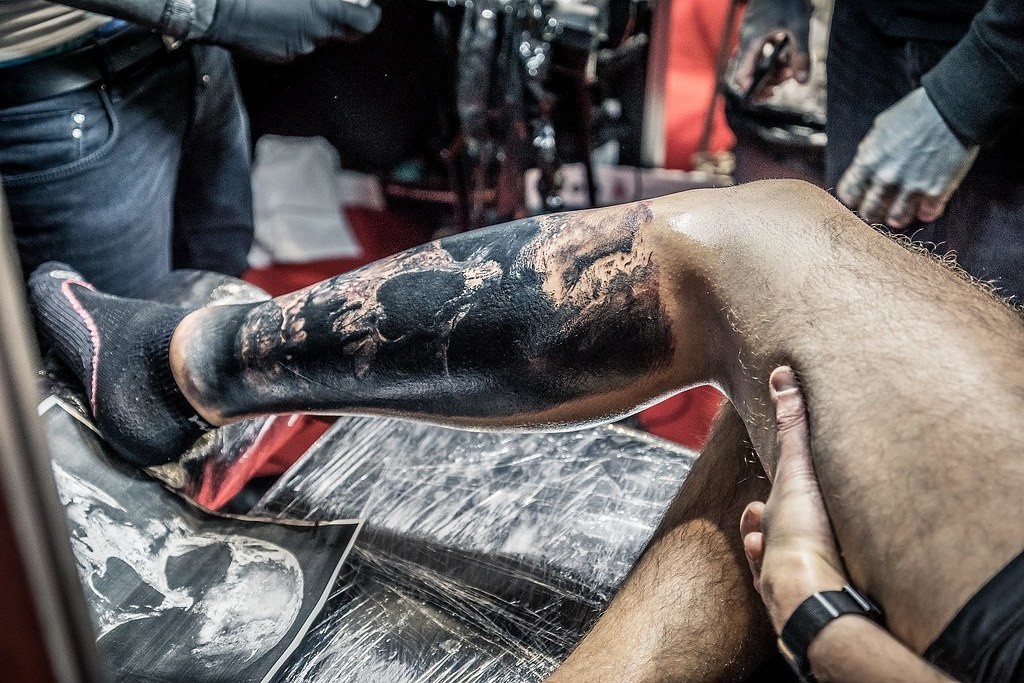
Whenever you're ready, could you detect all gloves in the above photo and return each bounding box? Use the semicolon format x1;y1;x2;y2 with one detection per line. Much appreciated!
192;3;380;61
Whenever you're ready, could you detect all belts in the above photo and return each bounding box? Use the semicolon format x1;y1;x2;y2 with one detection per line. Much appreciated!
2;24;173;109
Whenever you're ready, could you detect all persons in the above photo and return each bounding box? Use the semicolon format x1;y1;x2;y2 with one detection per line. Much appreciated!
726;2;1024;312
31;178;1024;683
0;0;383;300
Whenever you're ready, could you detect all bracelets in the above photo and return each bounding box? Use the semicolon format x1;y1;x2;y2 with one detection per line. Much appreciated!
156;0;197;42
776;585;881;683
